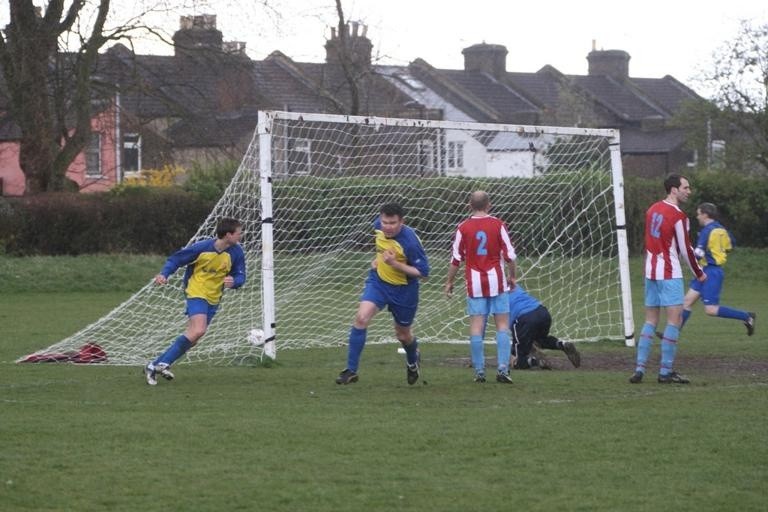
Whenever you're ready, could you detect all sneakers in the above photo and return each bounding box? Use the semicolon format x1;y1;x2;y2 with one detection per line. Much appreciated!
496;365;513;383
155;362;174;380
743;312;756;337
630;371;643;383
336;368;358;384
473;369;486;382
407;349;420;384
563;341;580;368
527;355;553;368
143;362;157;385
658;372;689;384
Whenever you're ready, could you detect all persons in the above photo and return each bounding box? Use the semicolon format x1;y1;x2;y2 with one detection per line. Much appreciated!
507;283;583;374
653;200;758;344
628;173;710;386
445;188;520;388
141;216;248;389
335;202;430;387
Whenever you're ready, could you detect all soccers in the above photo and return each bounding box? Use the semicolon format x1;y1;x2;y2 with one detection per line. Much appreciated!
248;327;267;349
